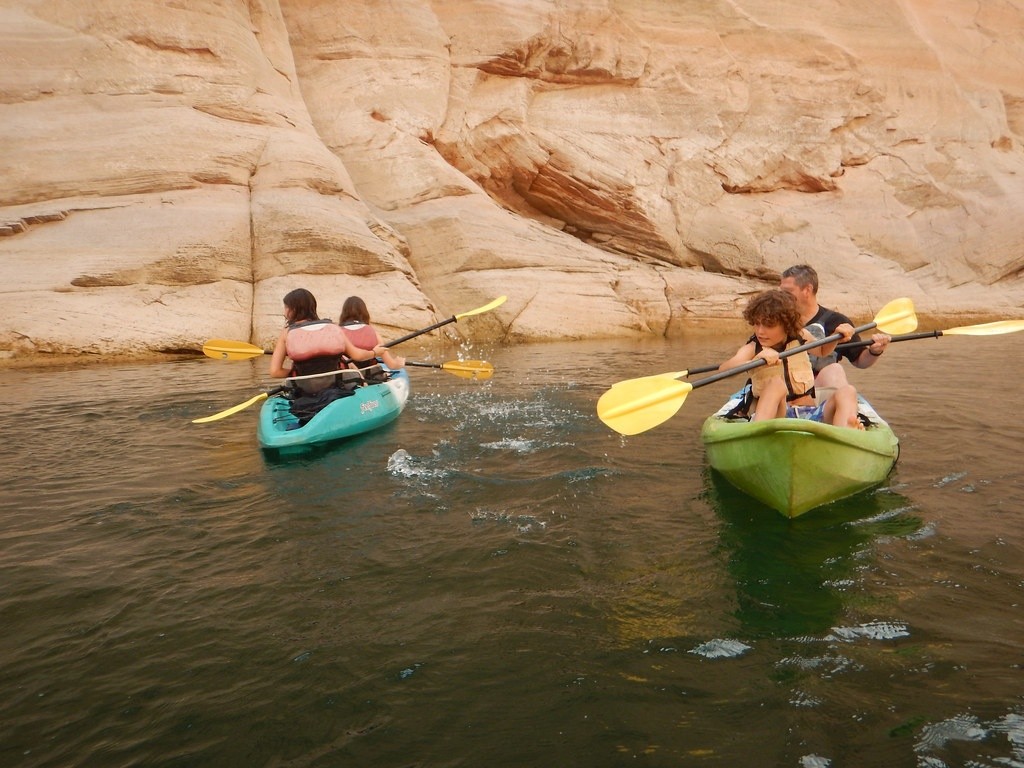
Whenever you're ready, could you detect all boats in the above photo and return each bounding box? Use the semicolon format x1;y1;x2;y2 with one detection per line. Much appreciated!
256;362;409;460
700;381;902;524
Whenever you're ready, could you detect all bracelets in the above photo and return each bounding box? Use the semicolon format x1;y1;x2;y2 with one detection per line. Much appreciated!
370;350;376;360
868;347;883;356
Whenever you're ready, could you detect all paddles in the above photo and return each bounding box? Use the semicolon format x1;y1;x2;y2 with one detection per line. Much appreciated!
611;319;1024;390
191;295;507;424
203;339;494;379
595;297;918;436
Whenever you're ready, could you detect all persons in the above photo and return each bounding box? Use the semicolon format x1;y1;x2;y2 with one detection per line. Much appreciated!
270;288;389;388
339;296;405;387
778;265;891;379
718;290;866;431
